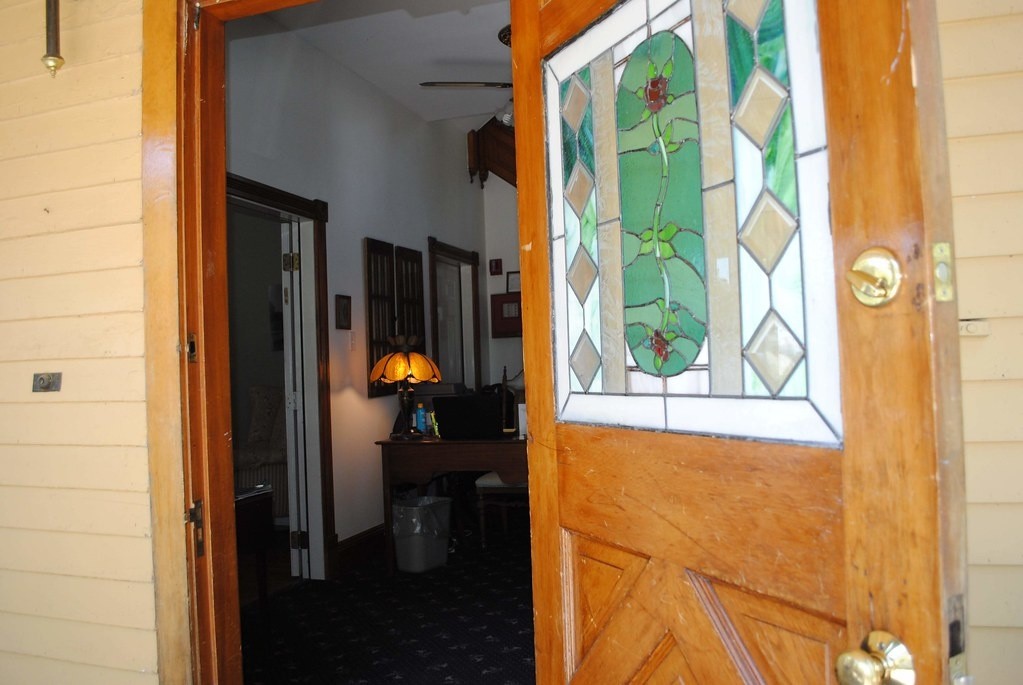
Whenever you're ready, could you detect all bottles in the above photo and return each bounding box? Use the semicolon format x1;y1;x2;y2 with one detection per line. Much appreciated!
417;403;425;434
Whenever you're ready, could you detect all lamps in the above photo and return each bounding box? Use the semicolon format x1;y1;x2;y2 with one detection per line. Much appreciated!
369;346;443;440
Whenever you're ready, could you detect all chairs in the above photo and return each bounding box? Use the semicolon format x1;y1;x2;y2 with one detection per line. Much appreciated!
474;400;529;549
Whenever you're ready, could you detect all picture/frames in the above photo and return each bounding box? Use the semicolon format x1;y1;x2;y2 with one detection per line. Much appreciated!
334;294;352;330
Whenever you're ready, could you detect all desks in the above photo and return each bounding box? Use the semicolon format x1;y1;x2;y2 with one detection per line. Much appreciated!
372;435;528;539
233;494;275;606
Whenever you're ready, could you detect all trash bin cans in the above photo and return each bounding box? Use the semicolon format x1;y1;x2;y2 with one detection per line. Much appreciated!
392;496;453;573
